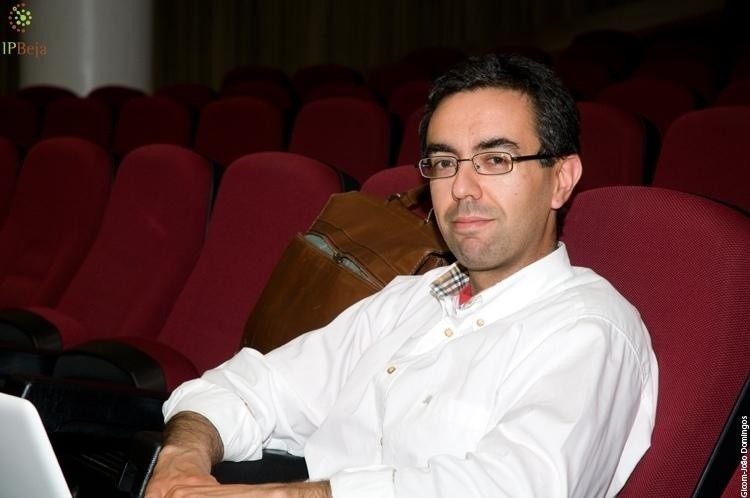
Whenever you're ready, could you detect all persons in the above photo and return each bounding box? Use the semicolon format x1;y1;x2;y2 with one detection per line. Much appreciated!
141;48;662;498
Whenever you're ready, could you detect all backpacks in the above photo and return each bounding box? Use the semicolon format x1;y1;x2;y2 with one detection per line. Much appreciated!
237;185;446;360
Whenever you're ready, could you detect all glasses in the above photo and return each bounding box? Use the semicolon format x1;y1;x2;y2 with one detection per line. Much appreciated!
418;152;549;181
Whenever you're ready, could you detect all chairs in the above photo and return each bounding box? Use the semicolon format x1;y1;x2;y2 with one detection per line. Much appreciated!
0;3;750;496
